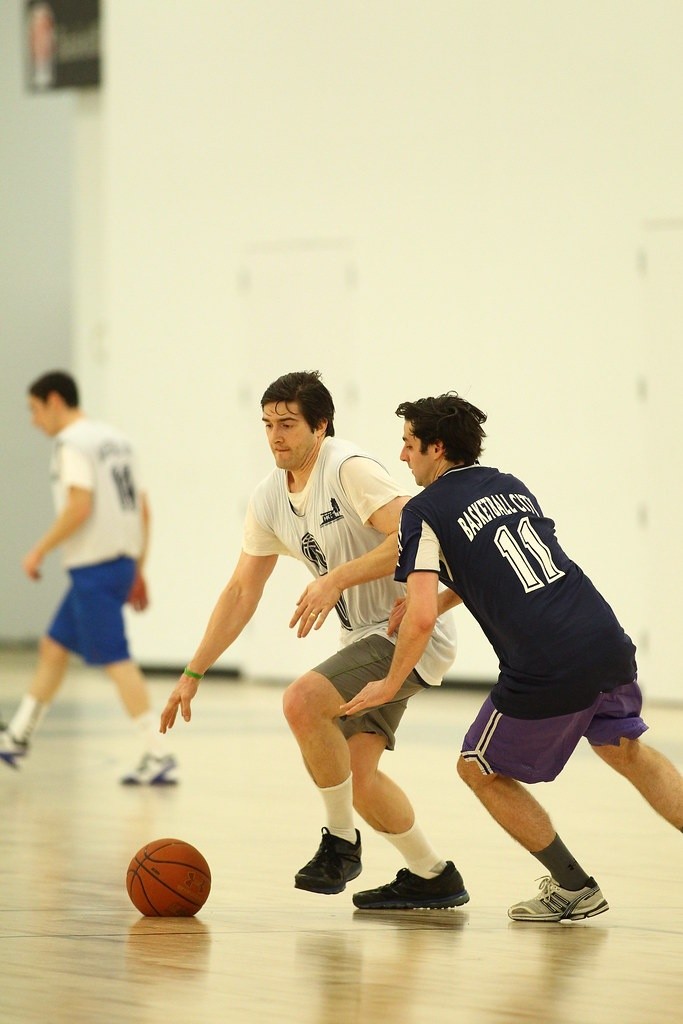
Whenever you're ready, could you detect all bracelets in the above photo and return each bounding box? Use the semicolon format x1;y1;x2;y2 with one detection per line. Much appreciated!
183;668;204;679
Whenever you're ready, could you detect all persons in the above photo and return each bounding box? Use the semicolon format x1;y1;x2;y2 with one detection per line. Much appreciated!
339;391;682;921
159;370;470;909
0;371;180;785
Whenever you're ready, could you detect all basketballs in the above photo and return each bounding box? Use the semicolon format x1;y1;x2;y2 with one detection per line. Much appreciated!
125;837;214;917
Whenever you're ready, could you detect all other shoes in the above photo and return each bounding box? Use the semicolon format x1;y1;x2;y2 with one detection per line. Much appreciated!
0;723;28;769
118;752;179;785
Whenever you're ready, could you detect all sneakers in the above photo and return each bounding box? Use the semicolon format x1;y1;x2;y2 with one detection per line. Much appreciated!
351;860;470;909
509;874;609;921
294;827;363;894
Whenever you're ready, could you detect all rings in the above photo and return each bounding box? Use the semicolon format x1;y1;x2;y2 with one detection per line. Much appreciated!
311;613;315;617
